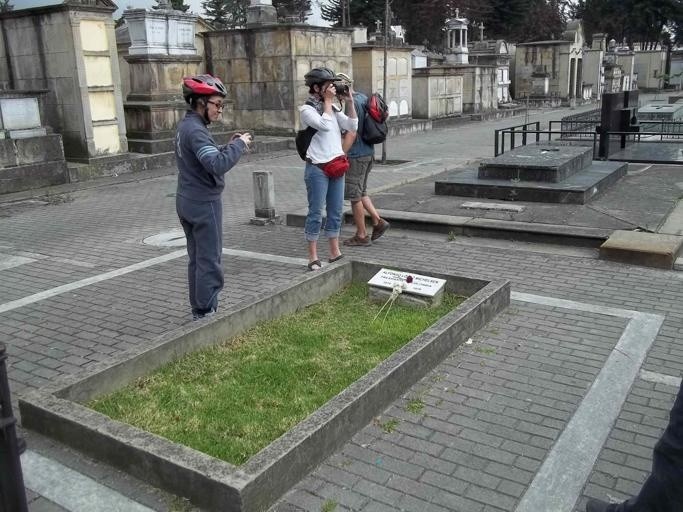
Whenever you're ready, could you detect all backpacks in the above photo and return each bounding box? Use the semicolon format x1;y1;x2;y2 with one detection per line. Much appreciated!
362;92;389;144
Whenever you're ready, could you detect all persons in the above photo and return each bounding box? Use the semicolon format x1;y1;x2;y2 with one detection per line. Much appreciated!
587;380;683;511
298;67;360;272
335;73;390;247
174;74;251;321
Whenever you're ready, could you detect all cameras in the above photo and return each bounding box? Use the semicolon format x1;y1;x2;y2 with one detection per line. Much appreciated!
330;82;349;97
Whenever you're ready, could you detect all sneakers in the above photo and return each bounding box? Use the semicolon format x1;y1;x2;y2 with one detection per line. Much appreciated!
307;260;322;270
371;218;390;241
344;234;370;247
329;253;345;264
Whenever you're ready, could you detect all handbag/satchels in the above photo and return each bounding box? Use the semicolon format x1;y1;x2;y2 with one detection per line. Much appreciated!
296;127;316;161
321;155;351;176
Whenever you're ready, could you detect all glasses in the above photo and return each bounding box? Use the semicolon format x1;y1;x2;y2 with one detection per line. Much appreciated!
199;96;225;109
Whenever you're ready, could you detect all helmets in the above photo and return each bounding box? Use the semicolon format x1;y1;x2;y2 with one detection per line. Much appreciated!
182;74;228;103
304;67;341;86
